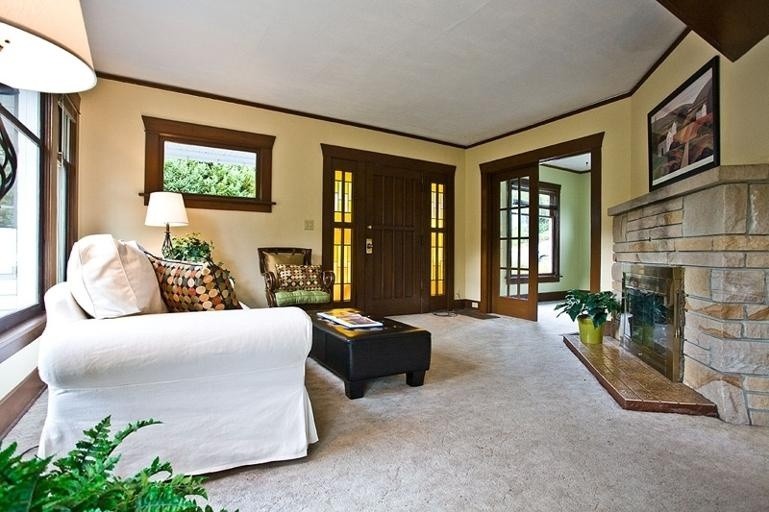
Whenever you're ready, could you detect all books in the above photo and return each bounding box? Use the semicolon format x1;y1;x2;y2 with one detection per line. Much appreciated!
333;314;384;329
317;309;356;323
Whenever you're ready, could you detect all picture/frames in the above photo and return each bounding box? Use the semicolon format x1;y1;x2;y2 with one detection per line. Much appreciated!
137;114;276;213
647;54;719;192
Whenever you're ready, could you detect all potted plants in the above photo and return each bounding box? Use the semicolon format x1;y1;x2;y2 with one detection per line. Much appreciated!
553;288;620;344
626;289;672;345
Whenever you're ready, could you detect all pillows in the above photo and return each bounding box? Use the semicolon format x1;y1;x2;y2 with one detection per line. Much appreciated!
274;263;322;292
143;251;242;310
66;233;168;318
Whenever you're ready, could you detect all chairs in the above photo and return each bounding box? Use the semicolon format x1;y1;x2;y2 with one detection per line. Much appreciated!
35;282;319;486
258;248;335;312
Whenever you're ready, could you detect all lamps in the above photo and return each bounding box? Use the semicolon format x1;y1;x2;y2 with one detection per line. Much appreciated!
144;191;189;254
0;0;95;200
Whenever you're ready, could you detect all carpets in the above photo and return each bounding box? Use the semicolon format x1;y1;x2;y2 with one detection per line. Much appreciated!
450;308;500;319
